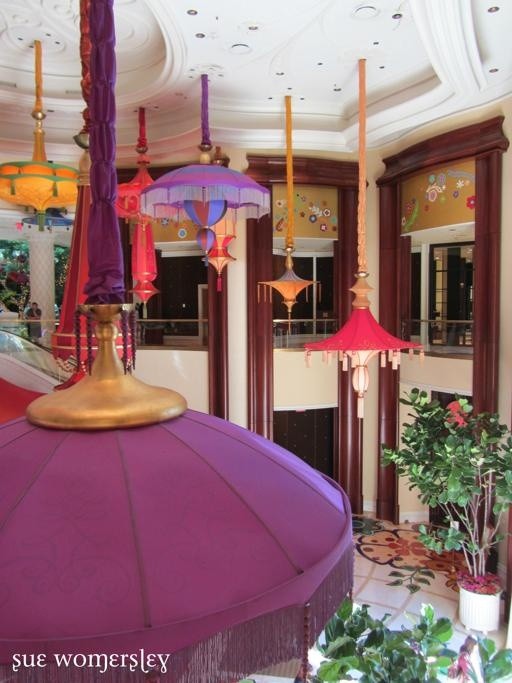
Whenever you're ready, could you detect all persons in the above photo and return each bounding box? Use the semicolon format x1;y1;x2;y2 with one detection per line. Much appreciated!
24;301;42;341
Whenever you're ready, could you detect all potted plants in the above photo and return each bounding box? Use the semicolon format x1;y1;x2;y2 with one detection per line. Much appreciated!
378;384;512;636
